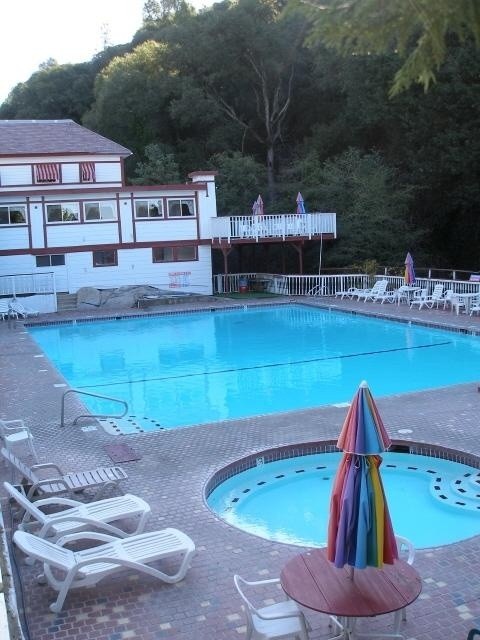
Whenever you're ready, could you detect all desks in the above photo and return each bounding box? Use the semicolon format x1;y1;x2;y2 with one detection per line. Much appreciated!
279;546;422;640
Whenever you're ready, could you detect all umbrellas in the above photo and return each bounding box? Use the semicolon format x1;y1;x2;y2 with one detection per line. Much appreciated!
258;194;263;215
327;382;397;578
252;201;259;214
404;251;416;308
297;193;305;214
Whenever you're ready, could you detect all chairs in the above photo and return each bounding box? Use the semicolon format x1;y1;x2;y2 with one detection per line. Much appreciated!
233;575;312;640
0;301;41;324
338;534;416;627
235;213;480;318
0;448;129;521
3;482;152;566
11;527;197;614
0;416;40;466
